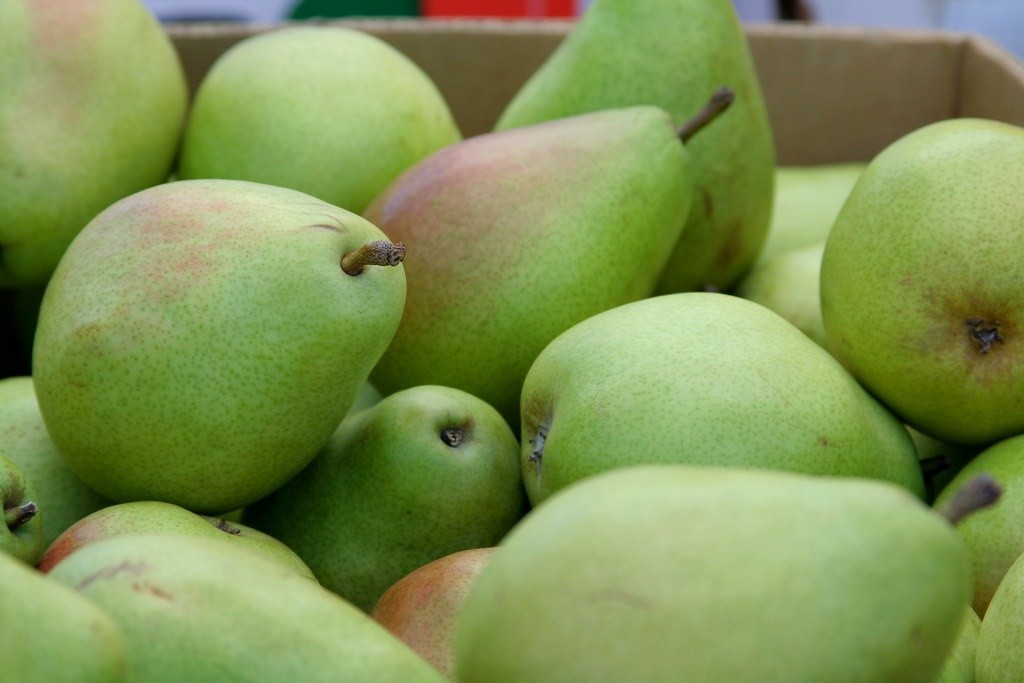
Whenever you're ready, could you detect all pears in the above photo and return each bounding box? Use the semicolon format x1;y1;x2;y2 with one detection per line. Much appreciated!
0;0;1024;683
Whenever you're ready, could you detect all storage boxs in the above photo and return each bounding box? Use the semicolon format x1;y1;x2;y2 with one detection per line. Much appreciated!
170;21;1024;164
